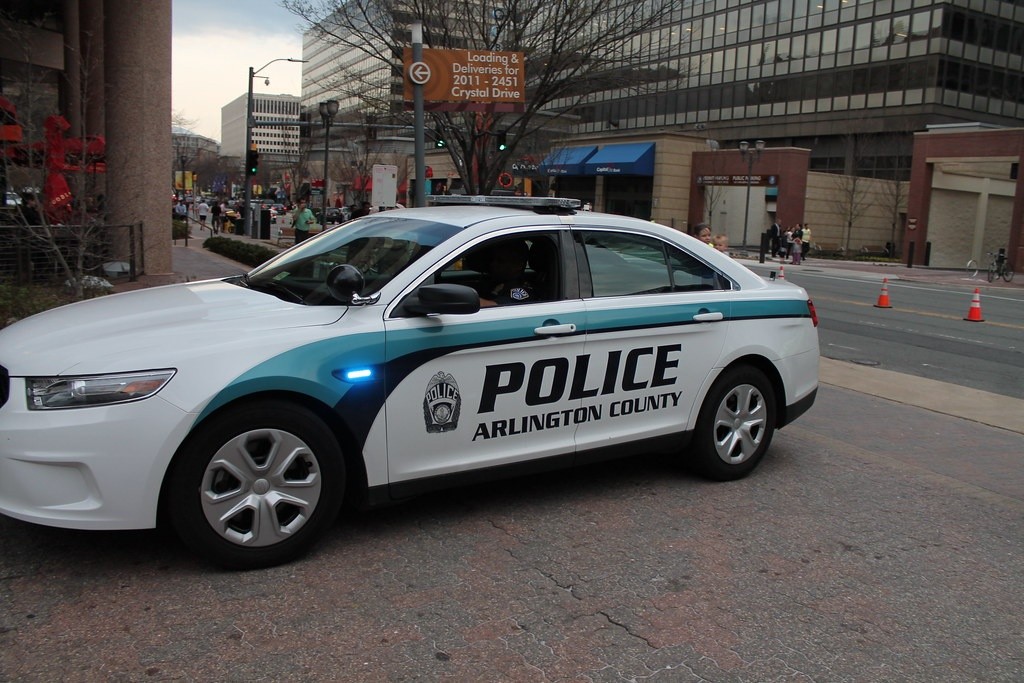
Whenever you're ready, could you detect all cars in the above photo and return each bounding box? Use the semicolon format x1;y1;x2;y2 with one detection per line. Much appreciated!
315;206;352;224
0;192;823;577
196;196;287;224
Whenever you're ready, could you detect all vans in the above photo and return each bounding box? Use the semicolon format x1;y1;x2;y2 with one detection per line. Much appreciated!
366;203;406;215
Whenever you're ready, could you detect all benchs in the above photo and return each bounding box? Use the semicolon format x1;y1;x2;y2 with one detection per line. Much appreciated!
277;228;319;245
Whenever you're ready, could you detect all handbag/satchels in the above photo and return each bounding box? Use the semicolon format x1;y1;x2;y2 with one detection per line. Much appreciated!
221;217;228;223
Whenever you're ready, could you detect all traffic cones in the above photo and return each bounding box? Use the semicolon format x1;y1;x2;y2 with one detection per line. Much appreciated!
963;288;985;322
873;277;893;308
777;266;785;280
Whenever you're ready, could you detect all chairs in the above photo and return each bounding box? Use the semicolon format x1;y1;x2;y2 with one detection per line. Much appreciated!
452;249;490;297
528;239;555;301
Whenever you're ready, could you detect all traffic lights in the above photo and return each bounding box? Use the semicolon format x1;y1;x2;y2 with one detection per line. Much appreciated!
246;150;258;175
496;130;507;151
435;126;447;149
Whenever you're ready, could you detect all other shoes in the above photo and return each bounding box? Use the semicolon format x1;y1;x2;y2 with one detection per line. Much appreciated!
214;229;218;234
802;258;806;260
772;254;777;257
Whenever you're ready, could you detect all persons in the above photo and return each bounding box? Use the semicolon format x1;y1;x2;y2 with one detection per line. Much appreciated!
478;239;548;308
694;222;729;257
175;198;225;235
771;217;812;265
293;197;315;244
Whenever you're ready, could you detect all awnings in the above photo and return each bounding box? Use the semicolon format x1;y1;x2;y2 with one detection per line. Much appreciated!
538;146;598;175
584;141;656;176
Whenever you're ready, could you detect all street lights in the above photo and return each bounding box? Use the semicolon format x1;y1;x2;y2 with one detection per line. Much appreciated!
179;154;188;204
739;140;766;247
318;99;340;231
244;58;309;236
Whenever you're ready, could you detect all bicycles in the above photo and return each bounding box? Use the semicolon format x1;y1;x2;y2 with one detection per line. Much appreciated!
986;251;1015;282
